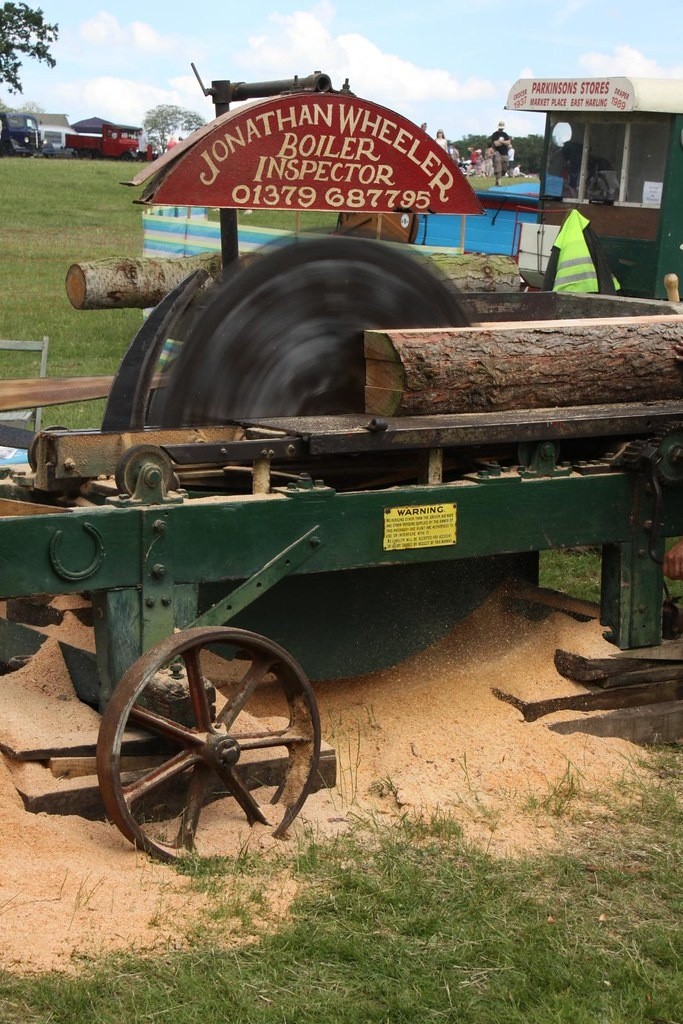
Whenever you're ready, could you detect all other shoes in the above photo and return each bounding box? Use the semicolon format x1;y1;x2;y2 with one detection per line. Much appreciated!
495;182;502;186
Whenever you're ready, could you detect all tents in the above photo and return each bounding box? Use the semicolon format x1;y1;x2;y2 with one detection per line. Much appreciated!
71;117;113;134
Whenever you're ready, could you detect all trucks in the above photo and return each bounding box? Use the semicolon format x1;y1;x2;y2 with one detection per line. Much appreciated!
64;124;143;162
0;111;42;157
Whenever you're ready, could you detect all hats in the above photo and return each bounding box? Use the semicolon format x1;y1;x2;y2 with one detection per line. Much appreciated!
475;149;482;152
498;121;505;129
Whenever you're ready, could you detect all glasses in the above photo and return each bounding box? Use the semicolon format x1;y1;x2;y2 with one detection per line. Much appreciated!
438;132;442;134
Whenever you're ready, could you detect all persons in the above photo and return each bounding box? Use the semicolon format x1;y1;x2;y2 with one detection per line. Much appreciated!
514;164;521;178
508;144;515;178
136;127;149;162
466;142;493;178
435;129;448;152
491;121;511;185
448;143;459;161
420;122;427;131
168;136;176;150
